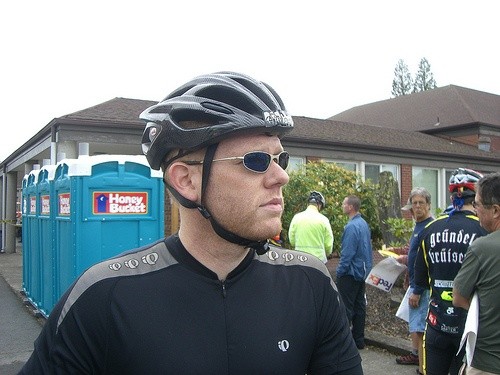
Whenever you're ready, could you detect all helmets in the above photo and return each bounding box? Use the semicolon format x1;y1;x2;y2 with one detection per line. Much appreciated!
139;70;295;170
448;167;484;192
307;191;325;209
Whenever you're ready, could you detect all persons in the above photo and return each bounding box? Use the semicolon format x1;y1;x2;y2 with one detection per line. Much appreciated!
333;196;372;349
17;72;363;374
385;168;500;375
288;191;334;264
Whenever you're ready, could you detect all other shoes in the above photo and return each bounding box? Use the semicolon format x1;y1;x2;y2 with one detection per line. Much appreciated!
355;337;365;348
396;351;419;363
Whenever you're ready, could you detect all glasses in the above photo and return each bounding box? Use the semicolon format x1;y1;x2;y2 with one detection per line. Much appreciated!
216;151;291;172
470;200;479;209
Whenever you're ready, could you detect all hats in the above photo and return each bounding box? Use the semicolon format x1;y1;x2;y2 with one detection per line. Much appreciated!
401;198;413;210
